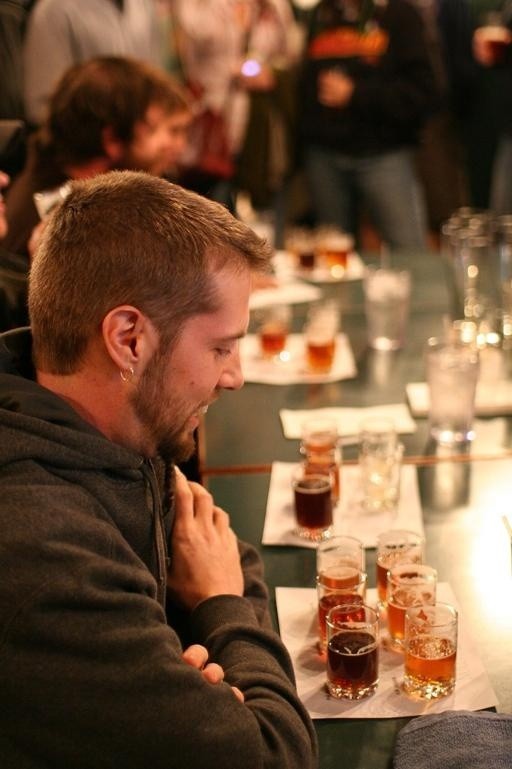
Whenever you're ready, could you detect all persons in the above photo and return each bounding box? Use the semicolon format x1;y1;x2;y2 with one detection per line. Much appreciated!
2;166;324;769
1;1;510;329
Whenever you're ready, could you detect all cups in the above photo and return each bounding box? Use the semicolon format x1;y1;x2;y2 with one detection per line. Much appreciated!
292;427;339;537
296;320;335;375
426;343;478;439
316;530;456;701
357;425;404;512
256;310;289;354
362;262;410;353
